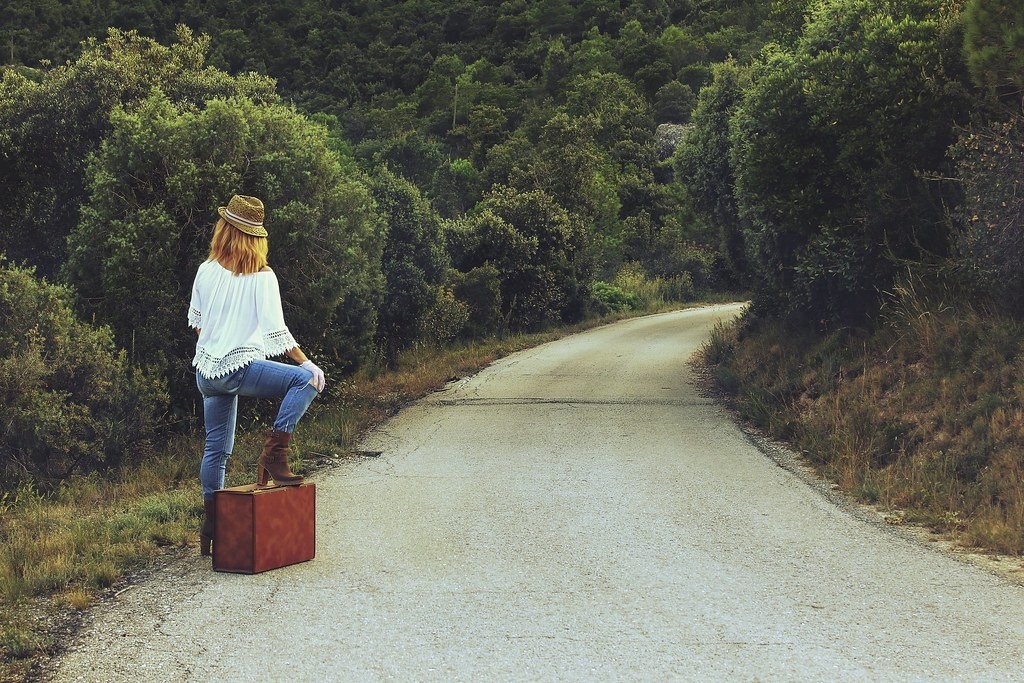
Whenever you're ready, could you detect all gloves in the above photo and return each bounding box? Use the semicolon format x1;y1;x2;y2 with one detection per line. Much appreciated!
298;360;326;392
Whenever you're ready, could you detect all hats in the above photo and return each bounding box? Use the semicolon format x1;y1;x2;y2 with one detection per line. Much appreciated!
216;194;268;237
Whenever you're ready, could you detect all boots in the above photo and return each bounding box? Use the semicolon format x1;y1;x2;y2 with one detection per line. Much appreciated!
199;497;214;556
257;431;304;487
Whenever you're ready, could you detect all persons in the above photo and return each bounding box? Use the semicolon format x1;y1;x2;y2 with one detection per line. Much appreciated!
188;195;325;554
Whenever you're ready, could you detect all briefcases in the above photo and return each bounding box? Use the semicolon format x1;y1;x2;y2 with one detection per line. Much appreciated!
213;481;316;574
